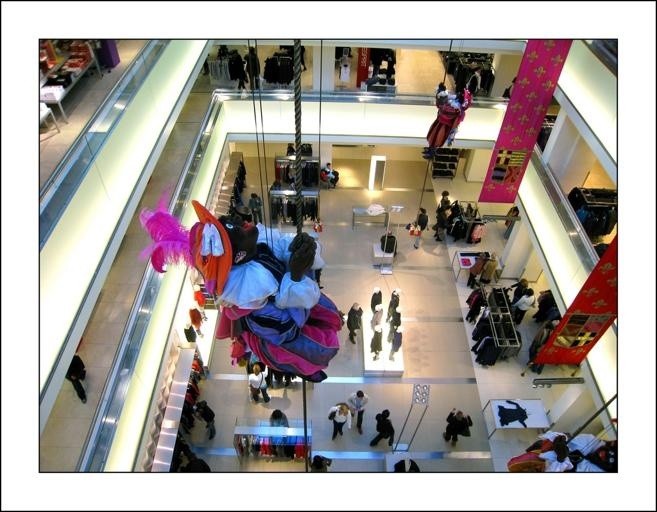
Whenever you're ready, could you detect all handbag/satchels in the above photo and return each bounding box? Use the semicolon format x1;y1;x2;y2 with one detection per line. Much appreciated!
251;388;260;395
409;224;421;236
328;412;336;421
460;423;471;437
389;437;393;446
406;223;411;230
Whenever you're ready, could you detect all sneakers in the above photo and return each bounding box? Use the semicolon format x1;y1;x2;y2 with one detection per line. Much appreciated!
205;424;209;428
208;435;213;439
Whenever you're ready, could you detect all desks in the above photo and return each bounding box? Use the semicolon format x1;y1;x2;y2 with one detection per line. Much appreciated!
352;206;389;230
482;399;550;439
452;251;482;281
40;51;102;134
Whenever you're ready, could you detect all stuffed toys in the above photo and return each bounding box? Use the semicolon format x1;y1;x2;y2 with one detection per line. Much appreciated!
179;201;347;384
421;82;472;160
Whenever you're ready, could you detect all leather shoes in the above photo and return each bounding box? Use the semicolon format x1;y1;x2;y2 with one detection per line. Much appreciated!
357;427;362;434
351;339;355;344
443;432;449;441
451;440;455;446
348;333;355;336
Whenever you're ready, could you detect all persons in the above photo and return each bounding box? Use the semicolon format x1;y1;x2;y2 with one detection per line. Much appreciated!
247;364;271;403
412;208;430;249
433;190;475;241
230;49;248;90
453;58;482;95
321;168;334;188
327;404;352;441
502;76;517;99
380;229;397;257
467;253;486;289
507;430;603;473
286;143;296;155
65;354;86;404
243;46;261;90
511;288;537;325
368;409;395;447
247;192;263;225
479;252;498;285
269;410;289;427
324;163;339;186
311;455;333;473
509;278;529;305
185;452;212;473
443;408;473;446
346;302;364;344
369;286;404;361
346;390;369;435
313;217;323;233
193;400;216;440
